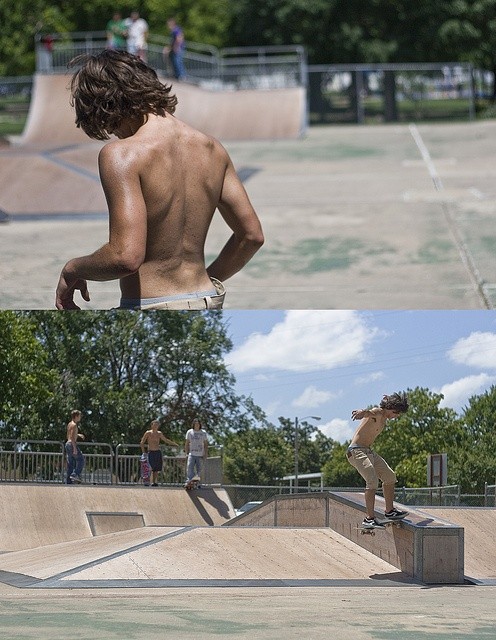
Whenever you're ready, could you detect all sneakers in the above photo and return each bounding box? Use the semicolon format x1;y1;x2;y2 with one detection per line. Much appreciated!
362;516;385;529
384;507;410;519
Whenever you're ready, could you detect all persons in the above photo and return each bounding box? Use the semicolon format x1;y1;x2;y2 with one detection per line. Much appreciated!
122;8;150;63
53;46;265;310
345;391;411;530
162;18;189;83
105;9;130;50
64;409;86;484
140;420;179;486
184;418;209;490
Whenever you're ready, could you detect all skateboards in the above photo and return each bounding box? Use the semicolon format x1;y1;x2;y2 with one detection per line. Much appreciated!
353;517;403;536
139;459;150;486
70;476;81;485
184;480;199;490
